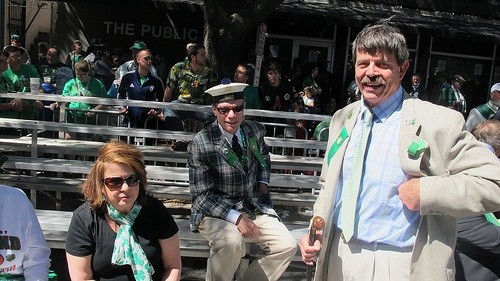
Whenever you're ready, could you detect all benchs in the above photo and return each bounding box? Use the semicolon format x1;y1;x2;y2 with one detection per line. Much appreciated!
0;92;332;278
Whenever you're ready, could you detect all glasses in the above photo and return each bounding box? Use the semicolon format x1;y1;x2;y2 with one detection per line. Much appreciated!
139;57;152;61
214;103;245;114
104;174;140;189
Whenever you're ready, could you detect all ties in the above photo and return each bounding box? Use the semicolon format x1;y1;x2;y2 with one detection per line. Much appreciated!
341;109;374;243
456;90;460;98
232;135;243;161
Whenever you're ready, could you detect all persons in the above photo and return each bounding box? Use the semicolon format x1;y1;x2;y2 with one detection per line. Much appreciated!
225;63;265;110
117;48;163;146
347;81;361;105
65;140;181;281
187;83;298;281
299;24;500;281
440;75;466;119
454;120;500;281
257;61;337;174
115;41;158;95
0;185;51;281
163;44;221;130
0;35;107;142
466;83;500;134
407;74;428;101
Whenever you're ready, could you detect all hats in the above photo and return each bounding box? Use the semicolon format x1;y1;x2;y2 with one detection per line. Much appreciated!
2;46;29;64
455;75;465;83
130;41;146;50
11;34;20;40
491;83;500;92
204;78;249;103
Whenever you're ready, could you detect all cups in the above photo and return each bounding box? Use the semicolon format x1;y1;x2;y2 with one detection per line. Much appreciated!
30;78;40;95
